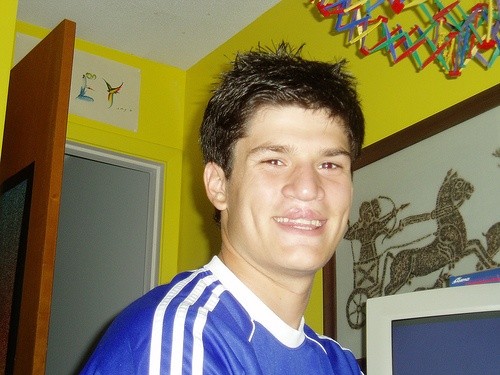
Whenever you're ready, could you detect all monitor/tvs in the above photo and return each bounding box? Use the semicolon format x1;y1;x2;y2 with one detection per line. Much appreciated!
367;282;499;375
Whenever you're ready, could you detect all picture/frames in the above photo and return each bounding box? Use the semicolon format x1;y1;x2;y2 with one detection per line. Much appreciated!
322;81;500;373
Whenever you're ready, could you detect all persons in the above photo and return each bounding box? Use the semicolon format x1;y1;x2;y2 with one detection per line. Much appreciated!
77;38;366;375
